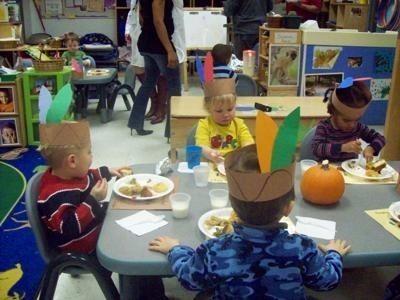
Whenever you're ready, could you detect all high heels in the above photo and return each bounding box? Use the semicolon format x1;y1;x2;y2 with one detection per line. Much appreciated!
131;128;153;135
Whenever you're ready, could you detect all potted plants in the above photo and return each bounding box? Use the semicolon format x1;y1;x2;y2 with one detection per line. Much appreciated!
267;5;302;29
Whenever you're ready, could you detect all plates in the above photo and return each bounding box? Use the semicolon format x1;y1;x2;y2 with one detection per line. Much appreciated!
113;173;175;200
390;200;400;221
198;208;295;239
341;158;395;180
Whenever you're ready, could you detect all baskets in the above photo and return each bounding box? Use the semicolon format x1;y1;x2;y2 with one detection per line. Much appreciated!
33;43;65;72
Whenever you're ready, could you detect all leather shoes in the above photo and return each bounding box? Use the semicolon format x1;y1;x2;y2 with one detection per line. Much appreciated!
150;112;165;124
145;110;155;120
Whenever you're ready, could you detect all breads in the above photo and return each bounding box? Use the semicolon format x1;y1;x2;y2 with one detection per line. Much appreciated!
372;160;386;172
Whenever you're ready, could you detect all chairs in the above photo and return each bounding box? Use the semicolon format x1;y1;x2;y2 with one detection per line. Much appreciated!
85;64;136;119
25;170;119;300
237;73;256;96
80;32;118;68
300;125;317;160
186;123;196;145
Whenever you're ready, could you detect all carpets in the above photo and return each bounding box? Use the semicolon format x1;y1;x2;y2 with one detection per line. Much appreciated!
0;144;49;300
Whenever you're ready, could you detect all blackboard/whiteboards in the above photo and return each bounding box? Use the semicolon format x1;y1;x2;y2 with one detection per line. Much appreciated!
115;7;130;46
183;8;227;50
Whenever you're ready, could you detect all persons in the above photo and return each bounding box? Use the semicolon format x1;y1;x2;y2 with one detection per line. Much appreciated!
62;32;92;118
211;43;239;86
0;89;14;112
36;83;169;300
194;51;254;160
120;0;185;135
1;123;18;144
275;0;323;30
222;0;273;61
384;273;400;300
312;76;386;163
147;106;351;300
279;49;298;85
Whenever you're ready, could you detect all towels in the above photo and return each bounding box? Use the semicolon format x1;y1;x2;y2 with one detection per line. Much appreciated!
115;210;168;236
178;162;208;173
295;216;336;240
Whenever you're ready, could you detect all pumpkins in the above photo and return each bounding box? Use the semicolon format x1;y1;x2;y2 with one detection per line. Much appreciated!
300;159;345;205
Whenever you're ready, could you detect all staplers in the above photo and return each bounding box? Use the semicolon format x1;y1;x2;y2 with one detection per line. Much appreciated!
255;102;271;112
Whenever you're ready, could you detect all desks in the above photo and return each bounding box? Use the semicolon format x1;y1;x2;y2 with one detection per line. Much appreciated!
300;28;397;134
0;43;82;69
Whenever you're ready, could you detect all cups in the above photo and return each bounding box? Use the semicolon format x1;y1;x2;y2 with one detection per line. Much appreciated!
243;50;255;75
301;159;318;173
170;193;191;219
186;146;209;187
209;188;229;208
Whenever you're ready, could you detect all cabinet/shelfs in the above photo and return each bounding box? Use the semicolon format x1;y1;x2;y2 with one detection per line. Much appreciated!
327;2;369;32
0;65;73;148
259;26;301;96
